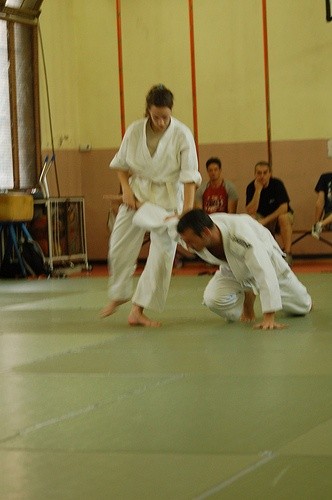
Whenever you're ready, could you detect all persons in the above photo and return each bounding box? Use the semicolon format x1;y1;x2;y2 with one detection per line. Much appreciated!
101;85;201;327
196;157;240;276
245;161;295;265
103;194;311;329
311;171;332;239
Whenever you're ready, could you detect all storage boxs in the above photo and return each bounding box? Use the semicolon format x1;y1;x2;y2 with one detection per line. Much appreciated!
10;196;94;281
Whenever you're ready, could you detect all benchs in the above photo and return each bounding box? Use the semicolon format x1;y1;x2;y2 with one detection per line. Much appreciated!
178;228;332;267
